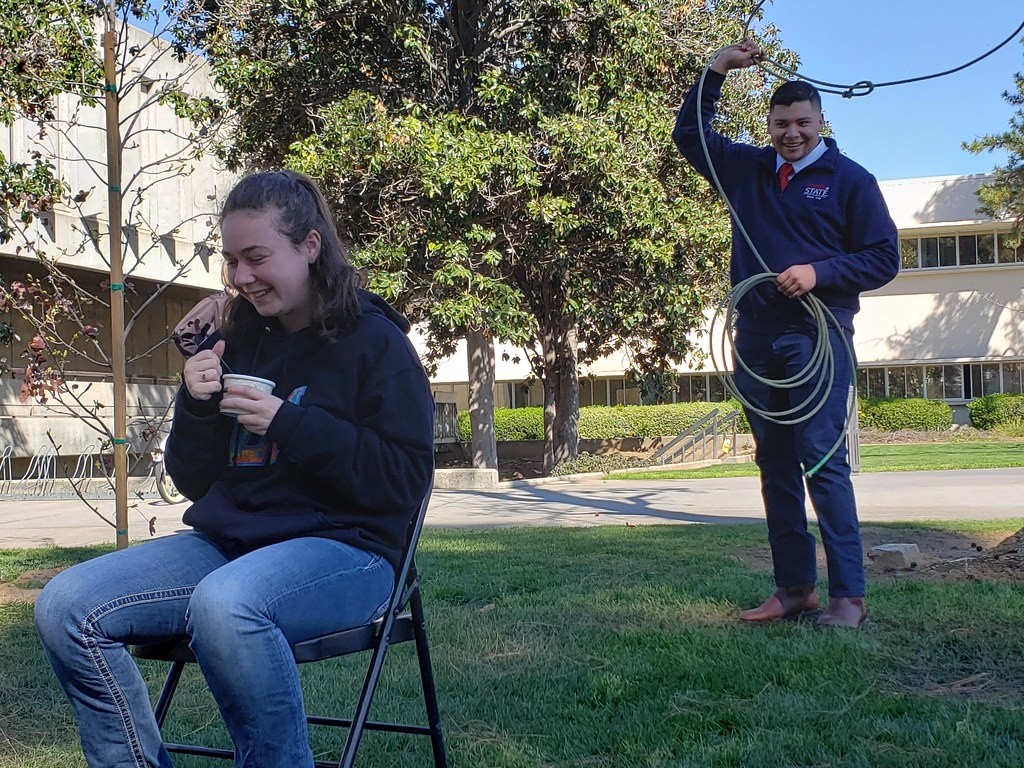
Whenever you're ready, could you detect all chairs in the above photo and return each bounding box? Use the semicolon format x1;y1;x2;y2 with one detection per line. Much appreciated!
153;453;445;768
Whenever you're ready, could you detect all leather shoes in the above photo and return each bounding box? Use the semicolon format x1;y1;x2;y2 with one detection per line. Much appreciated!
739;584;821;623
817;597;866;629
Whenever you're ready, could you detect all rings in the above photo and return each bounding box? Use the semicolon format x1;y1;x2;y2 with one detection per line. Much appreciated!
201;371;207;381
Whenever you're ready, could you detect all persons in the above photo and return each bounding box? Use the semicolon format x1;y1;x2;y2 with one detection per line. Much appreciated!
31;170;436;768
669;37;902;633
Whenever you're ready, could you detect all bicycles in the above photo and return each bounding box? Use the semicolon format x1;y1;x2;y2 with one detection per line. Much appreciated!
146;419;186;505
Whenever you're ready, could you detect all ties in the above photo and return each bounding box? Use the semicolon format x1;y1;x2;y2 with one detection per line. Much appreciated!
778;163;794;192
